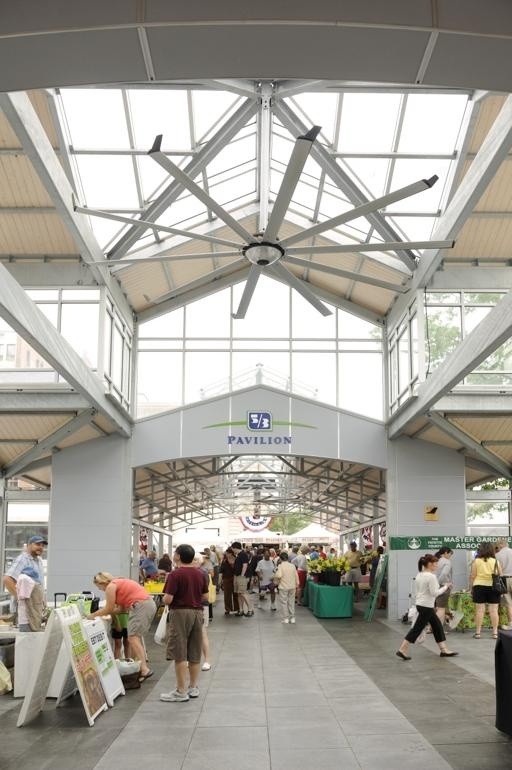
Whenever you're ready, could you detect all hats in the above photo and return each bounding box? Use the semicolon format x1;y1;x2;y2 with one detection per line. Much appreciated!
349;541;356;545
28;535;48;545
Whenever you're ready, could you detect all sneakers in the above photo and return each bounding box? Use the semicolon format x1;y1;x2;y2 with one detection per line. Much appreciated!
188;684;200;698
201;662;211;670
289;617;295;624
246;589;307;611
281;618;289;624
160;688;189;702
225;611;254;617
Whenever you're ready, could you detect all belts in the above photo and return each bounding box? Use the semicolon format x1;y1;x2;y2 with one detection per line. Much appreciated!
170;605;204;610
504;574;512;579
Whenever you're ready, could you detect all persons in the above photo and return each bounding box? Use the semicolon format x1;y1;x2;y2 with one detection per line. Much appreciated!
109;577;132;661
395;554;459;660
86;572;158;683
426;546;454;634
3;536;46;630
495;539;512;631
190;552;211;671
468;542;502;639
139;541;388;624
159;544;209;702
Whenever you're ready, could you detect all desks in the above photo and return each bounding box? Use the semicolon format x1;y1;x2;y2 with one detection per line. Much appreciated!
46;599;107;610
13;615;125;698
148;593;165;626
495;630;512;738
447;591;509;633
304;582;354;619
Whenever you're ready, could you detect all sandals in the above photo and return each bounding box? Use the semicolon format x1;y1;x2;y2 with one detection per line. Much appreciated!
426;627;432;634
491;633;498;639
472;633;481;640
139;670;153;683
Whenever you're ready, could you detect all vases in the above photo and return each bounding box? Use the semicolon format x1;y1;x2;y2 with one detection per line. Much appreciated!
313;573;322;583
327;572;341;585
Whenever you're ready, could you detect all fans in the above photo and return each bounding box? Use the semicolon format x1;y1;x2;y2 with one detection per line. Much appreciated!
70;82;456;319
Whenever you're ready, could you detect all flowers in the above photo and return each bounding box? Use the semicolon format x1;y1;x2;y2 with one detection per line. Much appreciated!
322;558;350;573
307;558;322;575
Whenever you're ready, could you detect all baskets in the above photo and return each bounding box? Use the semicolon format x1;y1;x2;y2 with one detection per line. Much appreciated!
62;591;100;618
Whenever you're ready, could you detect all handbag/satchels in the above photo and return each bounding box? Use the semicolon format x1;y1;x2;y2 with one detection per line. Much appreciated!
490;573;508;594
111;657;142;689
205;574;217;604
408;606;426;644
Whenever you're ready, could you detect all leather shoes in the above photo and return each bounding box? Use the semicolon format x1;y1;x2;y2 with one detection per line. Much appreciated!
440;650;459;656
395;650;411;660
